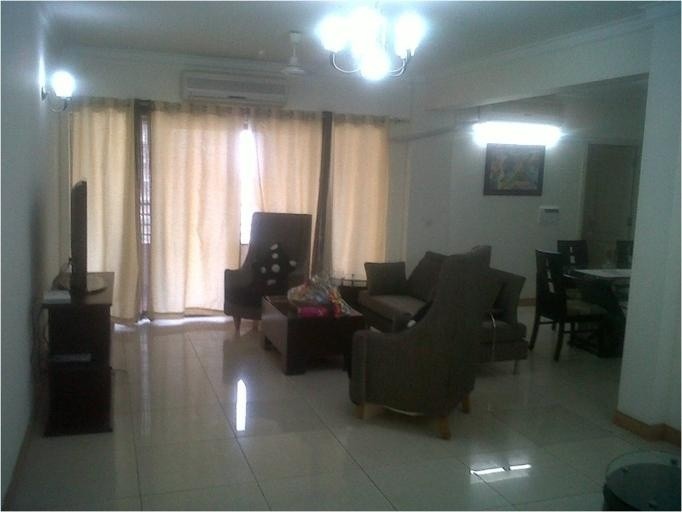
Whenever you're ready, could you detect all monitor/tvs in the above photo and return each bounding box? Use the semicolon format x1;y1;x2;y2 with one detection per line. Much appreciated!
57;180;107;303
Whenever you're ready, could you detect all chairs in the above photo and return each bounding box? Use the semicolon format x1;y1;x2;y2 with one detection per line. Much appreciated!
530;250;609;364
552;239;589;339
347;253;502;440
616;238;636;274
222;211;312;334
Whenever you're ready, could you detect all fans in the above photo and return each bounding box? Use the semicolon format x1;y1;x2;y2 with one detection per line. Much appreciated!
281;28;308;76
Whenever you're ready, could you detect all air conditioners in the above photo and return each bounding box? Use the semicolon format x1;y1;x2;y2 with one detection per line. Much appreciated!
177;69;289;108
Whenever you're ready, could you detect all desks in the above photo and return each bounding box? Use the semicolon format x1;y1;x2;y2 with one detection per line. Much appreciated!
563;268;635;360
600;447;681;511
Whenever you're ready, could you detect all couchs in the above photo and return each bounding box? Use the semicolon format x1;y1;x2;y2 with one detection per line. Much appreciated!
357;248;530;373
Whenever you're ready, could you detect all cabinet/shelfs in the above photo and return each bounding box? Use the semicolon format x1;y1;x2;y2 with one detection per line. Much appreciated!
40;270;117;439
337;286;367;304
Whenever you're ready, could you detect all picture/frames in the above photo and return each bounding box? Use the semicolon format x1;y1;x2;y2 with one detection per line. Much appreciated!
481;141;548;198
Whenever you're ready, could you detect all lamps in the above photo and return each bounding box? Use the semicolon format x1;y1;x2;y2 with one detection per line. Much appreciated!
317;1;428;84
41;68;76;114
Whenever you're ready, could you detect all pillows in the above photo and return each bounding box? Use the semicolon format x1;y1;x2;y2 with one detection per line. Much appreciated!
363;260;409;296
245;241;297;286
